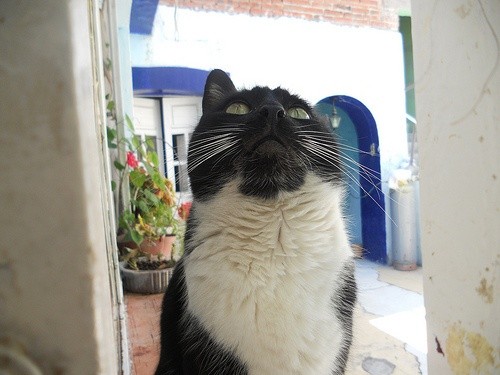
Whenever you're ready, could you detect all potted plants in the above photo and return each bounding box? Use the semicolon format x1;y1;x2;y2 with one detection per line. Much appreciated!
103;55;180;296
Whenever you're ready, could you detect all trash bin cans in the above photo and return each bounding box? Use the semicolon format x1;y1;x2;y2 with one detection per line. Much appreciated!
388;177;419;273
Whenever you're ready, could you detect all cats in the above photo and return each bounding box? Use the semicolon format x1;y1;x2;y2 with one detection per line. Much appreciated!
155;69;399;374
